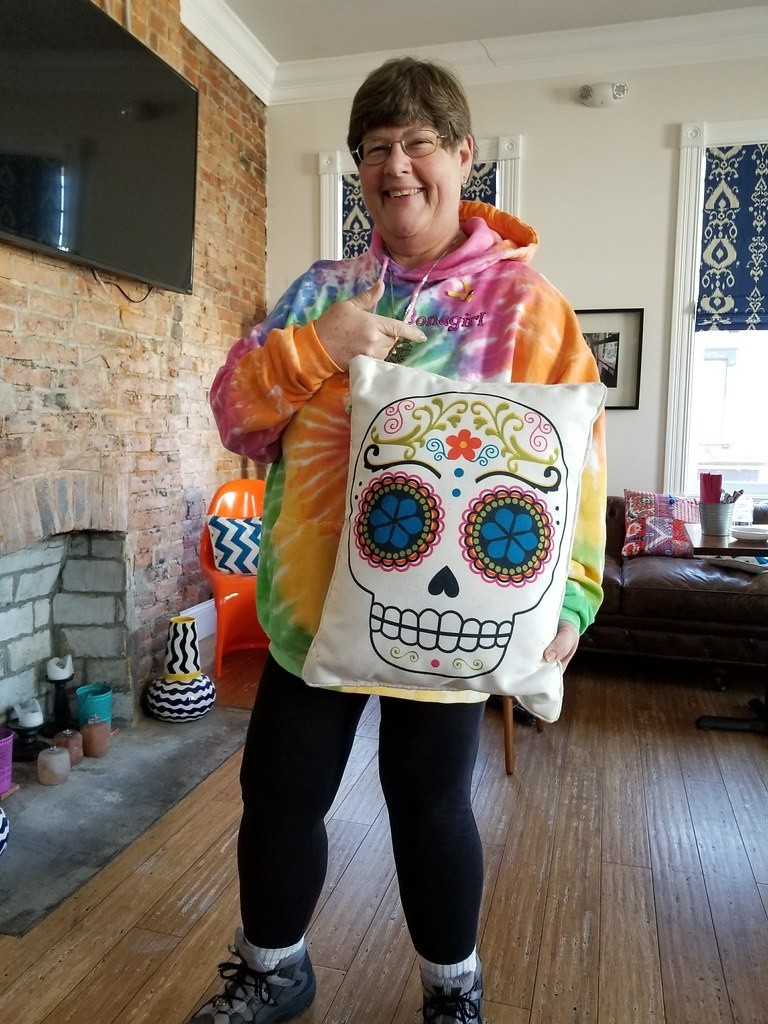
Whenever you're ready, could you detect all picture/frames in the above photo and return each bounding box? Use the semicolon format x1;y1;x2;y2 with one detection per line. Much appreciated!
572;308;645;411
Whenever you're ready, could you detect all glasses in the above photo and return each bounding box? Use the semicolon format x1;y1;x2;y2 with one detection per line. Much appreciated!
351;129;448;166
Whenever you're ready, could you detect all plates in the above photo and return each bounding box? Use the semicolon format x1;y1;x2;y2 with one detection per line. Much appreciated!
730;524;768;541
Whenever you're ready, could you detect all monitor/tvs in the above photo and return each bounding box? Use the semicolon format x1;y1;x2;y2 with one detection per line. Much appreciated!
0;0;199;293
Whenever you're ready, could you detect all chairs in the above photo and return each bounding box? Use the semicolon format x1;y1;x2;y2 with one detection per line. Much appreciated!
200;478;270;678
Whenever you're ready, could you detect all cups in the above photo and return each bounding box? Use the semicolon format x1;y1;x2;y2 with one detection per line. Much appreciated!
699;503;733;536
76;684;112;732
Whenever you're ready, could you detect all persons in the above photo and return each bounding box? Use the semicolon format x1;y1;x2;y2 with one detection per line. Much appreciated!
179;58;612;1024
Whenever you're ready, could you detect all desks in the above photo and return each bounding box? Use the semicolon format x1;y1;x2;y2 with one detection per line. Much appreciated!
684;524;768;736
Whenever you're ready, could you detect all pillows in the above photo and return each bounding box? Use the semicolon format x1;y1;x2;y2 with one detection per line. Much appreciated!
621;489;700;558
704;556;768;575
206;516;262;576
302;353;609;722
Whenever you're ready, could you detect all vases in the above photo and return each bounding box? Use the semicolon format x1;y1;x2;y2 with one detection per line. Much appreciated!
146;617;217;722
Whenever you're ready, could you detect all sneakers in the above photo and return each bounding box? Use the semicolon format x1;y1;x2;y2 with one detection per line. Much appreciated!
420;951;484;1024
188;927;317;1024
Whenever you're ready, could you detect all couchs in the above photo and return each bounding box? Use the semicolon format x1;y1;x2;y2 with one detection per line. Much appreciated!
569;495;768;680
